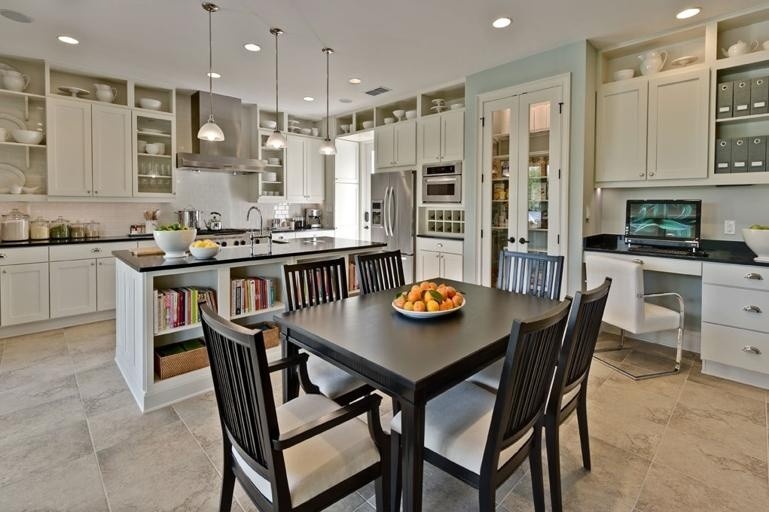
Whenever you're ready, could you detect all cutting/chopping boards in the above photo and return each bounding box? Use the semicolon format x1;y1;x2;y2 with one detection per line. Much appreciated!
129;247;161;256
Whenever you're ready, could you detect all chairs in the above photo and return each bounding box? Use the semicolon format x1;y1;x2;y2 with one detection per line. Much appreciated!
585;255;684;379
466;251;564;395
391;296;575;512
355;247;401;297
466;277;613;510
198;301;390;512
281;257;379;411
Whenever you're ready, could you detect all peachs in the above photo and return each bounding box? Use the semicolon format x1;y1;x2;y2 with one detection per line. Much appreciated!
394;281;463;312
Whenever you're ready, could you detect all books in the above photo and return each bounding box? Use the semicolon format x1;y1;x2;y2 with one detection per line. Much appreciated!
349;261;359;290
154;287;217;333
231;277;277;315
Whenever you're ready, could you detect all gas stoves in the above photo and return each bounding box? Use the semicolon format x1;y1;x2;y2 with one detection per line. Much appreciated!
197;228;247;235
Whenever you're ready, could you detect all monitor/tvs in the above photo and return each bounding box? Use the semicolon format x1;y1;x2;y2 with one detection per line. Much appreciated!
625;200;701;248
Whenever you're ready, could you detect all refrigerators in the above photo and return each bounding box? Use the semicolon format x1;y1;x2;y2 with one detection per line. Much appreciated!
370;170;414;255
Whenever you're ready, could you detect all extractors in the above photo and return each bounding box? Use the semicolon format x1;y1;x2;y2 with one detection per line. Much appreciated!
176;91;264;176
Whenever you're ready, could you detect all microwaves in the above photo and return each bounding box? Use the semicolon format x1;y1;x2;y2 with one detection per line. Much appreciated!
421;161;463;203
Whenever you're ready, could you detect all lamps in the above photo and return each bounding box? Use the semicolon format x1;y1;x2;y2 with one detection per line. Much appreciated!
675;7;701;21
264;27;290;151
198;1;225;142
318;47;339;156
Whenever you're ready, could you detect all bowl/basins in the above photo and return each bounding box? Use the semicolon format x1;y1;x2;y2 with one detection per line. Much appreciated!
742;228;769;262
140;98;161;110
189;247;220;259
12;128;43;144
263;172;276;181
154;229;197;258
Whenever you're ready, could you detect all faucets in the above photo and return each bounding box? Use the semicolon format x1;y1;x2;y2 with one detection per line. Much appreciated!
246;206;264;235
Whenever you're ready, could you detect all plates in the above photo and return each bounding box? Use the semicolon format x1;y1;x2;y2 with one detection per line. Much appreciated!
392;292;466;319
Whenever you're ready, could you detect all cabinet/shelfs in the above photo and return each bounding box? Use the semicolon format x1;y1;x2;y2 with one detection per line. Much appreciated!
586;234;769;390
418;108;466;163
712;7;769;185
416;165;464;283
0;244;50;338
333;130;373;240
593;20;713;189
373;121;418;170
111;237;387;414
50;237;139;333
0;53;176;201
475;74;571;297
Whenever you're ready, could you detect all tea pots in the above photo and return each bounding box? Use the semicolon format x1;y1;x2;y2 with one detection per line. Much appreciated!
720;39;760;58
1;68;31;92
203;212;222;231
94;83;117;103
636;48;668;76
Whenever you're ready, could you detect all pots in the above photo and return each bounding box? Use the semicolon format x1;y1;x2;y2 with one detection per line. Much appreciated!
175;206;203;231
